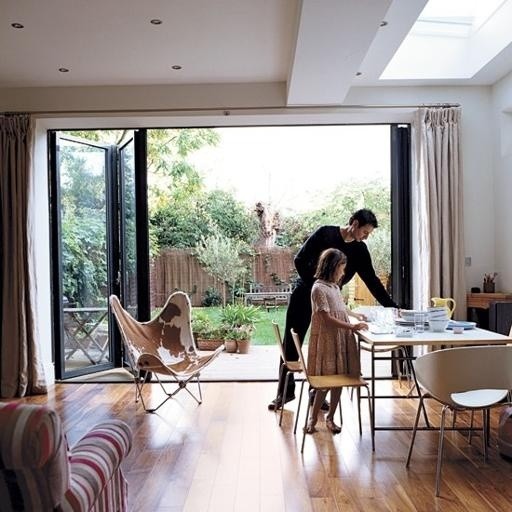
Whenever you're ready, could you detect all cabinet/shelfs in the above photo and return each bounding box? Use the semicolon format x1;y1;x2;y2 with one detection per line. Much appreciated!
468;290;510;335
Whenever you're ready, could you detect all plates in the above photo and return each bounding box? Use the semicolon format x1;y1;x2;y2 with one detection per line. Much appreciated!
445;321;477;330
396;318;429;327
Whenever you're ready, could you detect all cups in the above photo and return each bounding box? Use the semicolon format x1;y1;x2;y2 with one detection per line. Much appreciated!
414;314;425;333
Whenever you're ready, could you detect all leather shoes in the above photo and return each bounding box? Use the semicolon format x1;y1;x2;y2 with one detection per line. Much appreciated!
269;391;295;410
310;398;330;410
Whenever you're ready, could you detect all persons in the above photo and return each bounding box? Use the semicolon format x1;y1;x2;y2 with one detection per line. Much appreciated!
270;210;398;432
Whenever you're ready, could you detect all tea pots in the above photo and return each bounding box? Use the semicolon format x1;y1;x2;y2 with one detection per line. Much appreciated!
431;297;456;320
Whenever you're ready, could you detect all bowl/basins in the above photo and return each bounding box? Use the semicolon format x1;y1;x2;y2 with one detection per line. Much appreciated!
453;326;465;334
400;310;428;321
426;309;449;332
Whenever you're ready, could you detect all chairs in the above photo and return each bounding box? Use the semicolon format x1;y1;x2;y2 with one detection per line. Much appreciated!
264;298;280;312
348;303;432;433
108;290;225;412
272;319;345;429
288;327;377;453
2;404;133;512
402;341;511;497
